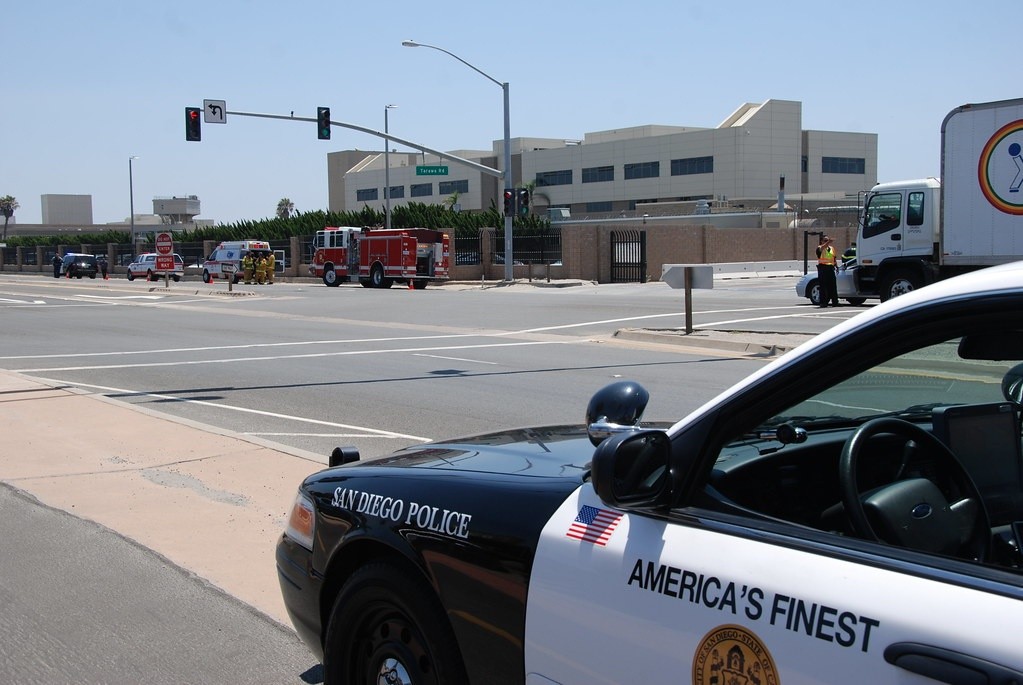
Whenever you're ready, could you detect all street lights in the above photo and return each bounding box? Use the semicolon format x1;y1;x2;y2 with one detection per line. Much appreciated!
402;41;515;280
65;272;69;279
129;154;141;259
384;105;398;228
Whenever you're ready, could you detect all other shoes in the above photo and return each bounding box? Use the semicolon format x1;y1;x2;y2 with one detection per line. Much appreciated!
832;303;843;307
820;304;827;307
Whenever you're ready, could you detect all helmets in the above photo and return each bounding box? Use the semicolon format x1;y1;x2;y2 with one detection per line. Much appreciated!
258;252;262;255
268;250;272;253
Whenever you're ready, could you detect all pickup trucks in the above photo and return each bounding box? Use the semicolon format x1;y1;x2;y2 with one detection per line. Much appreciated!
127;251;184;282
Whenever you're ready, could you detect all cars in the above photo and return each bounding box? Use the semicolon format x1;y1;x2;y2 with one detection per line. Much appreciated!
275;259;1022;685
456;252;522;265
796;254;867;305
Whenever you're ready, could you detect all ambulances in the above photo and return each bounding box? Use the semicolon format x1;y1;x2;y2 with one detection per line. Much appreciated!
201;239;286;283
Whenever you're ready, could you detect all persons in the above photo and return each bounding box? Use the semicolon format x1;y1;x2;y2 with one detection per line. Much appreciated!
891;198;918;220
242;251;275;285
841;241;857;265
100;258;108;279
815;235;844;308
51;252;63;278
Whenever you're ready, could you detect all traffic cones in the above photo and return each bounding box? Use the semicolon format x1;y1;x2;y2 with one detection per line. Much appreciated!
105;273;109;280
146;274;151;283
208;275;214;284
407;279;415;290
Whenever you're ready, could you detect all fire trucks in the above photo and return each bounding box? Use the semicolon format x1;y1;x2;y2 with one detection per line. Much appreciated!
310;225;451;289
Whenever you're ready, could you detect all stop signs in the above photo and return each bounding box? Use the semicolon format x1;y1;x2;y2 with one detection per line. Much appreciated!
154;232;173;256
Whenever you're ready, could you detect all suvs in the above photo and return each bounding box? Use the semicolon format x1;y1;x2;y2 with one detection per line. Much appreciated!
60;252;98;279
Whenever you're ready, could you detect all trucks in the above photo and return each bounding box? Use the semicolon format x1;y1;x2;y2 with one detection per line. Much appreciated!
854;98;1023;302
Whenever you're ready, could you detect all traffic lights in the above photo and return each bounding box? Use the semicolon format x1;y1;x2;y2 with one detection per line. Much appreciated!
518;187;530;215
316;107;331;139
186;107;202;141
503;188;516;218
